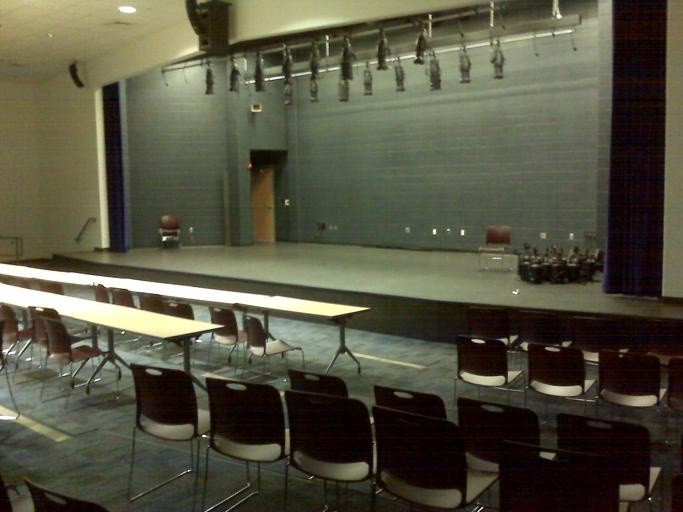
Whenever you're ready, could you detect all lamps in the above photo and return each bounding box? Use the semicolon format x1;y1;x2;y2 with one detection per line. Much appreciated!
201;3;508;105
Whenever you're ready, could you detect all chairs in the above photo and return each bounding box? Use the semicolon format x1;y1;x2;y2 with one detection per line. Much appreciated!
476;224;513;271
158;216;181;250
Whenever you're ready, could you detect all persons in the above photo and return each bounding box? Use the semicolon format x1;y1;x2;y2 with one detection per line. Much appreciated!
584;247;603;265
567;245;583;264
